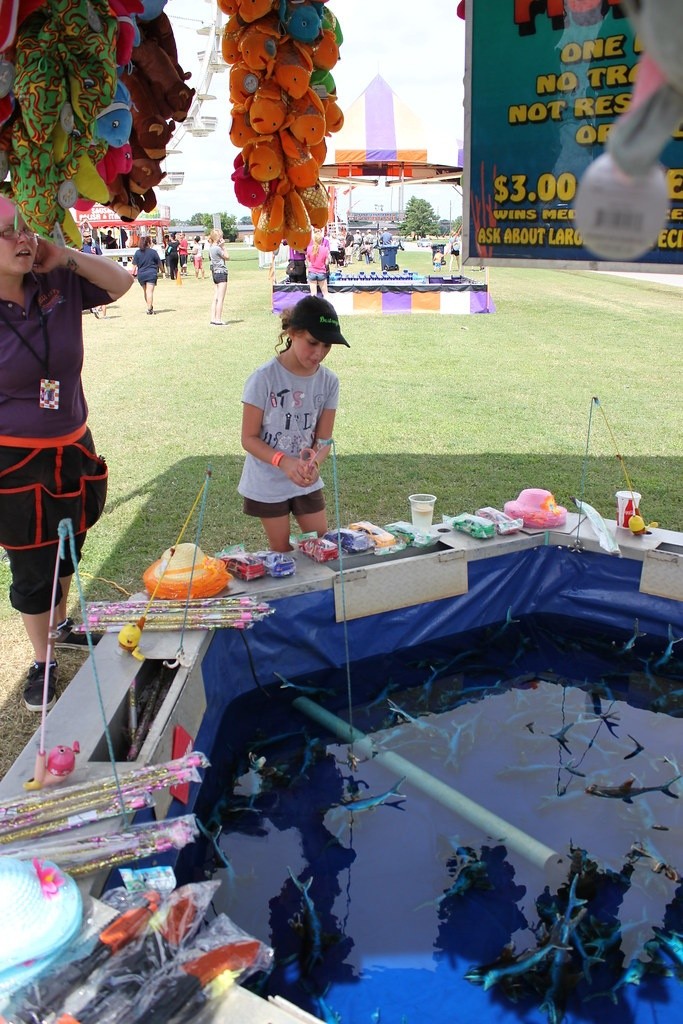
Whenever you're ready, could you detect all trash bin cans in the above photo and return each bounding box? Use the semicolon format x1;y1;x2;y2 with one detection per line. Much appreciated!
380;244;399;271
431;244;446;265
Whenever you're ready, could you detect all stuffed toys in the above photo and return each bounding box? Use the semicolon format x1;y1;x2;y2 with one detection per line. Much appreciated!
218;0;344;252
0;0;195;237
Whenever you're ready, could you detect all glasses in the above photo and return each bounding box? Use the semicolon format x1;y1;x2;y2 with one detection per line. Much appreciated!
0;226;35;240
84;235;91;238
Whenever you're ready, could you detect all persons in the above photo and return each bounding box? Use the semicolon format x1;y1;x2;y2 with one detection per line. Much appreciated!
0;184;134;716
82;227;463;280
306;233;331;300
93;305;108;319
237;295;351;553
131;236;165;314
208;229;229;325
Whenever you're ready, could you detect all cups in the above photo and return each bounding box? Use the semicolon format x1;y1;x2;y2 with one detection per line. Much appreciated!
408;493;437;530
615;491;641;530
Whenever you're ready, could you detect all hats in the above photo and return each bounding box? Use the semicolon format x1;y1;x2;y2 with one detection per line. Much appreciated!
384;227;388;231
504;488;567;529
356;230;360;232
143;544;233;599
0;854;82;999
289;295;350;347
367;229;371;233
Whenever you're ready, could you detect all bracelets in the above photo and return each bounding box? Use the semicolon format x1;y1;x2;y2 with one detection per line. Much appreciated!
272;451;285;469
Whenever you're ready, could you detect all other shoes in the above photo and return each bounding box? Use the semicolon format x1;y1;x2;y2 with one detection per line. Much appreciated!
181;273;187;276
211;322;227;325
147;308;156;314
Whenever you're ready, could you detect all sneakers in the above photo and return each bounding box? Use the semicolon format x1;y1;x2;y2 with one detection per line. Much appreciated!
53;618;103;651
23;661;57;711
90;308;100;319
102;316;111;320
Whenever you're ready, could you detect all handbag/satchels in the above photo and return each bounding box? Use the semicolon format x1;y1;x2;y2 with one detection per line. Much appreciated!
165;242;173;256
190;251;194;262
286;261;305;276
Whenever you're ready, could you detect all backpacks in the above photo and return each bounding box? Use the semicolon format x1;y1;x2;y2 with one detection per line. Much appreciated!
452;236;460;251
